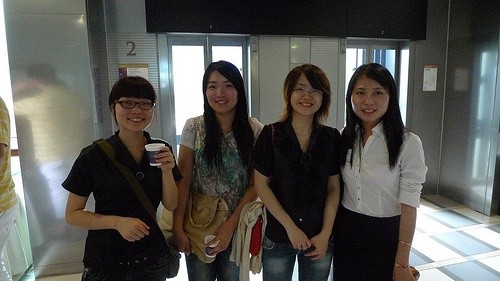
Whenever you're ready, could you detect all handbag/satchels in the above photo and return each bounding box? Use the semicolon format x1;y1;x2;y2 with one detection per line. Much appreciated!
166;241;181;278
157;191;229;263
248;215;264;256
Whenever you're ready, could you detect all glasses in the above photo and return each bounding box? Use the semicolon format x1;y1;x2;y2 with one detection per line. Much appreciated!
114;99;156;110
294;87;323;96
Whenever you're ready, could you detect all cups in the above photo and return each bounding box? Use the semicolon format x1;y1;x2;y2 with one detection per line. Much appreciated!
203;233;221;258
144;142;166;169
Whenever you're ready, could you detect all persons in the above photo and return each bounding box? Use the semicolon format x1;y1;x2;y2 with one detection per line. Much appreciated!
11;63;91;242
61;76;183;281
0;89;19;281
249;63;343;281
172;60;265;281
340;63;428;281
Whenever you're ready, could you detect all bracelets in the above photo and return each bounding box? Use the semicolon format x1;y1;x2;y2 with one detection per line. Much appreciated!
399;240;412;246
395;262;410;268
